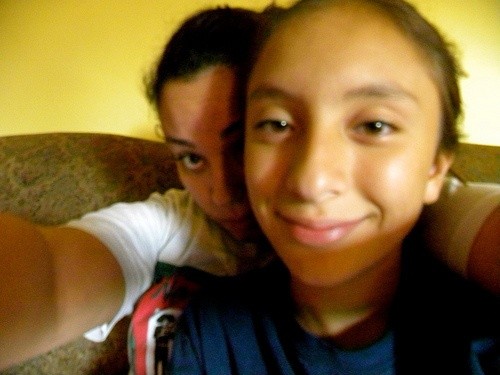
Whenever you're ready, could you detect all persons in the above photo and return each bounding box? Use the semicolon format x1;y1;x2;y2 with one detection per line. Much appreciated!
0;4;499;375
168;0;500;375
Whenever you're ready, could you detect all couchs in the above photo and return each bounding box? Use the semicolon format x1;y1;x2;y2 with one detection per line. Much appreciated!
0;134;500;374
